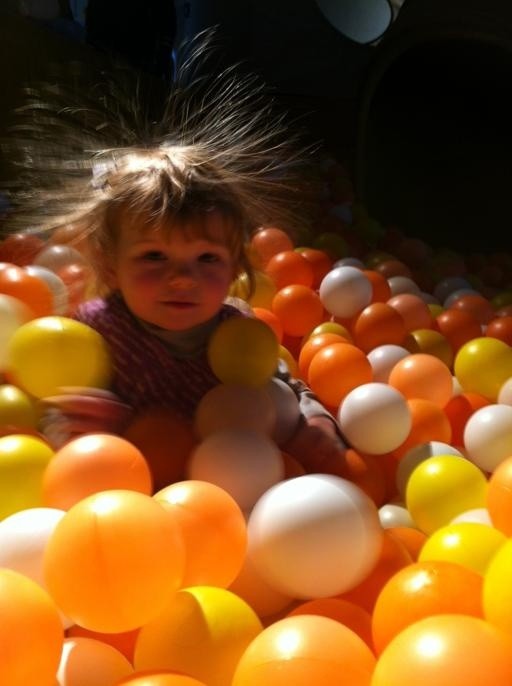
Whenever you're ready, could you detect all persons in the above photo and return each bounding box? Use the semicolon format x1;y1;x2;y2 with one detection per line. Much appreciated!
38;134;354;474
